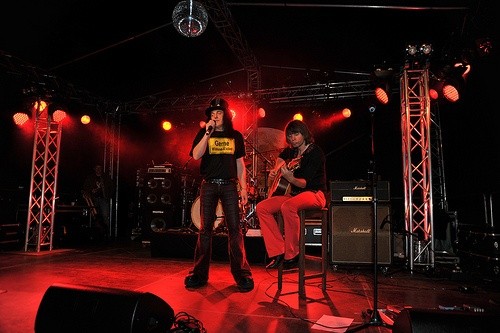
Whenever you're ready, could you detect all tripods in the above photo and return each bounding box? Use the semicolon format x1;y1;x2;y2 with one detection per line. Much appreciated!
238;140;273;229
170;157;197;233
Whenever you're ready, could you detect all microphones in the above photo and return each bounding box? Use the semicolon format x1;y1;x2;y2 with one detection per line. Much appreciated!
369;106;375;113
207;120;216;135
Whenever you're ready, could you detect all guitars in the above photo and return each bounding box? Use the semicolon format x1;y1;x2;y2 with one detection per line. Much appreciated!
268;161;300;199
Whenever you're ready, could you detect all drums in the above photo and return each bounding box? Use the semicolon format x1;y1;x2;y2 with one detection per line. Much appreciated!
191;195;246;232
237;179;258;199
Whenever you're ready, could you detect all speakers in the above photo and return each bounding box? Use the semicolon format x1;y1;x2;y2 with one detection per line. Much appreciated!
137;179;177;233
330;204;392;265
37;286;172;333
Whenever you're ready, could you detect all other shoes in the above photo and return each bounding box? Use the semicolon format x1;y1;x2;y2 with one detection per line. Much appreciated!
265;253;285;269
279;254;300;270
237;274;254;290
183;272;208;287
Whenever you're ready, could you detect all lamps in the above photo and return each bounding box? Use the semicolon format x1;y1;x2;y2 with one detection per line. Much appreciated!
173;0;208;37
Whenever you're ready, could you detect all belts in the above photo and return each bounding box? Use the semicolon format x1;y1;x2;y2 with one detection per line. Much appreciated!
202;178;238;184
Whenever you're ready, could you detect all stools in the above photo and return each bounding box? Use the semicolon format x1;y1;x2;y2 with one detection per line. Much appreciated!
277;210;326;295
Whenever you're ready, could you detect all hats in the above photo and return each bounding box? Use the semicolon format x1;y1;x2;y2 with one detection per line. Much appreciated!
205;99;233;119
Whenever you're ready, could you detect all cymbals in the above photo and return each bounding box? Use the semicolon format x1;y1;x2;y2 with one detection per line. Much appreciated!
187;175;199;184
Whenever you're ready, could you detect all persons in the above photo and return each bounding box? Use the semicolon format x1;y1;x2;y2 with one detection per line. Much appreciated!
257;119;328;271
81;165;113;241
184;98;254;292
